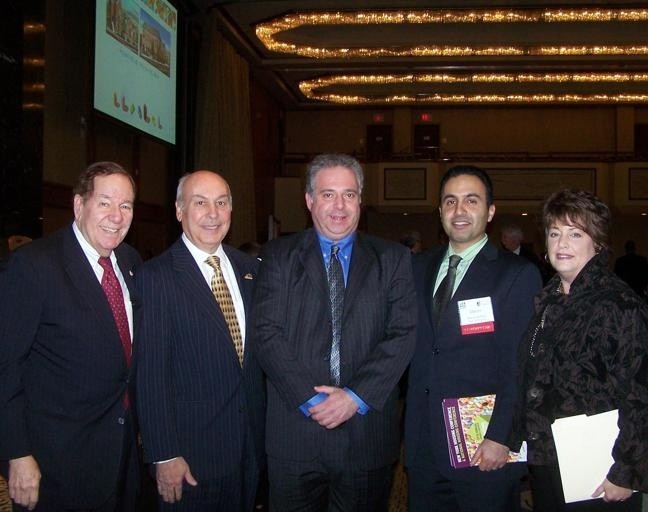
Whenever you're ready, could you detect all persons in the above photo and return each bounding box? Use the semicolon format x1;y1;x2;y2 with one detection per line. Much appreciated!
498;221;537;266
611;237;647;299
400;231;422;255
238;241;262;257
405;165;542;511
250;155;416;511
1;162;145;511
134;168;267;512
508;190;646;512
438;228;449;245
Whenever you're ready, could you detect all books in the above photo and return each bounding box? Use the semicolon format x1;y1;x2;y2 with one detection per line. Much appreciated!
439;392;530;468
548;408;641;503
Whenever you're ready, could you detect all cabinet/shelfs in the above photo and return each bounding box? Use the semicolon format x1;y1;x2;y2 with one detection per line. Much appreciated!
412;120;441;162
362;122;393;161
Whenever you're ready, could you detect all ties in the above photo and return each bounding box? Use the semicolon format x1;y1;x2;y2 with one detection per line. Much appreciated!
428;253;461;338
204;253;244;362
97;255;133;412
327;244;346;387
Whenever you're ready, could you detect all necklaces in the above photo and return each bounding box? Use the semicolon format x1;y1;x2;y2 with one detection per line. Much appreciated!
527;278;563;360
